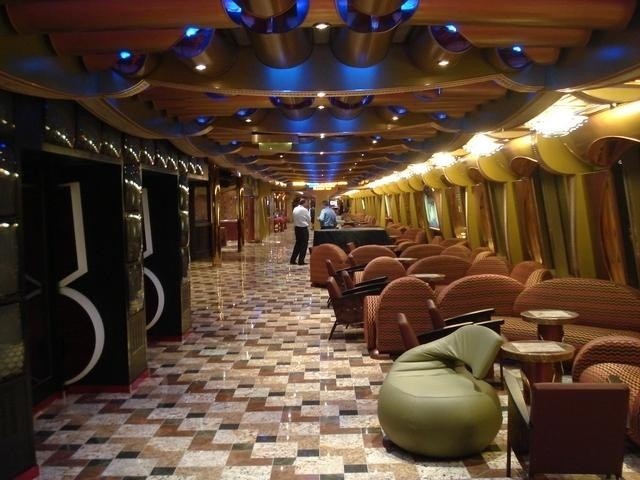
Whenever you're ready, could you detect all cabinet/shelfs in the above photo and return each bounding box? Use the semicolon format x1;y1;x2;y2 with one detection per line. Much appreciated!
219;220;237;241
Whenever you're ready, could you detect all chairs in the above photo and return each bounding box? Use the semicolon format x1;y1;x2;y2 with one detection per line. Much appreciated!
505;375;630;480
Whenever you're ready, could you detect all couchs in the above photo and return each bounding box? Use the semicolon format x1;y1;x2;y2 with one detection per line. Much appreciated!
310;214;550;356
573;335;640;447
505;278;640;357
378;326;503;459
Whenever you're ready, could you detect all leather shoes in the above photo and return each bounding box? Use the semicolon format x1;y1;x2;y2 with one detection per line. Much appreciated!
290;261;297;264
298;262;307;265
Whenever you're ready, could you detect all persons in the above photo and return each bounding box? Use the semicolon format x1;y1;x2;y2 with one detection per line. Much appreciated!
290;198;311;265
309;206;315;229
318;201;337;229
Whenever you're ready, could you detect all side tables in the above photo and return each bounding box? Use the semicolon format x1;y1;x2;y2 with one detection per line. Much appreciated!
521;308;579;342
502;340;574;404
